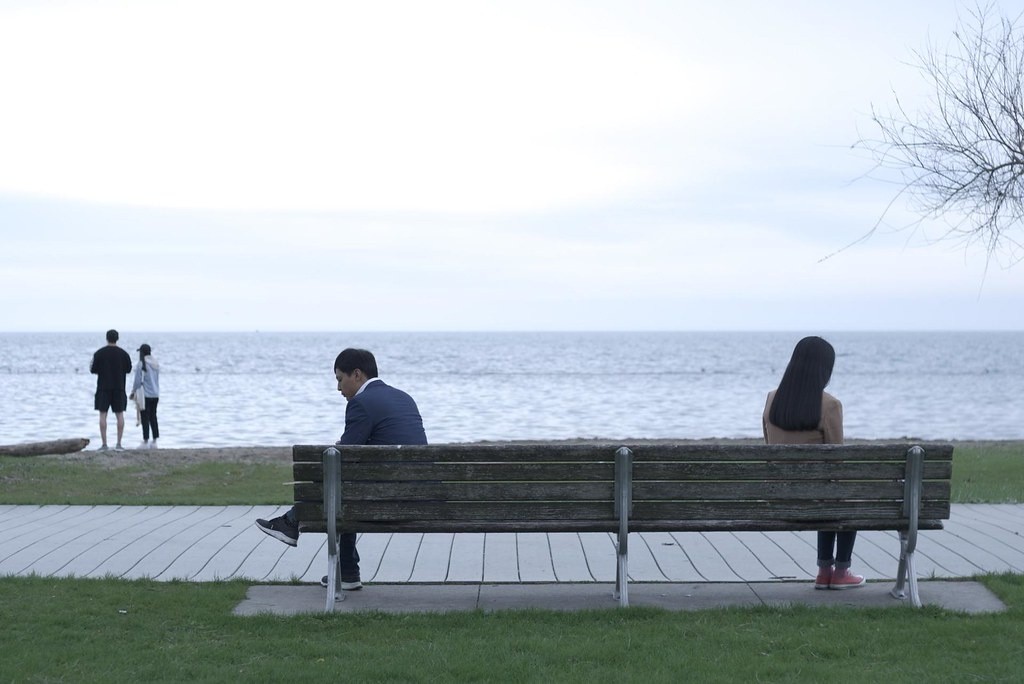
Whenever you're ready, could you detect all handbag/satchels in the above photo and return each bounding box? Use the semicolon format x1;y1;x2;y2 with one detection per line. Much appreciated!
134;386;146;411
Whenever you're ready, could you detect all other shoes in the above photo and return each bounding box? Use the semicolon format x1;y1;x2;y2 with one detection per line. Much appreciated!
150;443;158;450
98;444;109;451
114;447;125;452
137;444;148;450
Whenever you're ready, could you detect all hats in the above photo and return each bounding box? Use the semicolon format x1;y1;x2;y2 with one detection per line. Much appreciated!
136;344;151;352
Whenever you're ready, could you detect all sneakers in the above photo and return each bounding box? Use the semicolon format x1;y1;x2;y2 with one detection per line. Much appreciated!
255;517;299;547
321;575;363;591
815;566;866;590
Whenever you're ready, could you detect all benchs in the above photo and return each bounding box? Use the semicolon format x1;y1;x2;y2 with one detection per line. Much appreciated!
292;443;953;608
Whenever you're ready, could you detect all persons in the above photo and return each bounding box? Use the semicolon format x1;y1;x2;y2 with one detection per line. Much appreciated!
128;344;160;451
90;330;131;452
254;347;428;591
762;336;866;591
